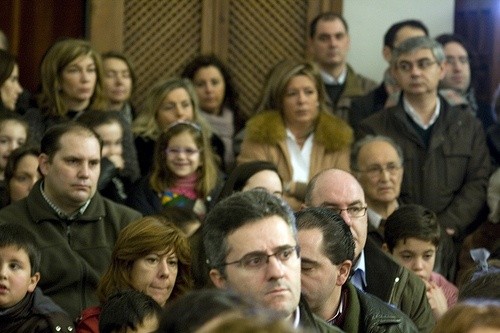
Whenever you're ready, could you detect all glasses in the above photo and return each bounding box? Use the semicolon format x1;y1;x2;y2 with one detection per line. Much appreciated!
394;58;436;72
212;244;298;271
354;163;401;179
333;206;369;218
166;146;203;158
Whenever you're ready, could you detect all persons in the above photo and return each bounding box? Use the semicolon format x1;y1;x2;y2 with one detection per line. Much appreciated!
0;12;500;333
